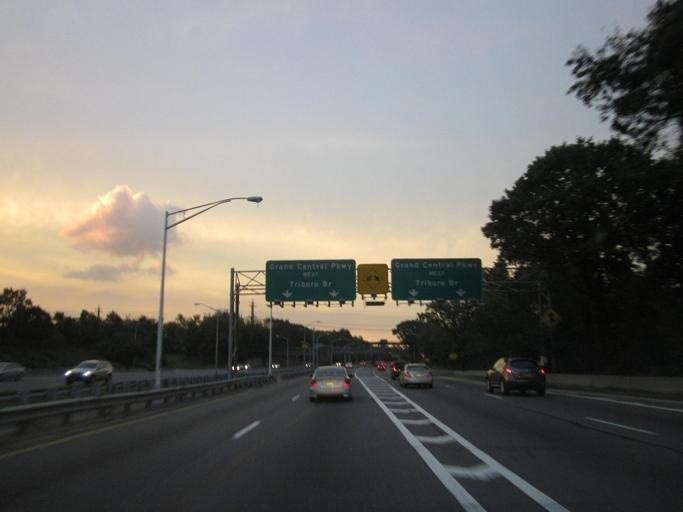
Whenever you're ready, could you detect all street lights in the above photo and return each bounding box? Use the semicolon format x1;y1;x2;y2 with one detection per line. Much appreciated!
133;321;143;343
153;195;263;390
276;334;289;369
193;302;220;370
303;320;343;369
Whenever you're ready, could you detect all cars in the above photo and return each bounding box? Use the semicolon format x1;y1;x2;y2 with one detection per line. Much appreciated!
305;360;368;402
232;363;250;370
0;362;27;382
272;364;281;369
374;359;433;389
485;356;546;397
65;359;114;386
